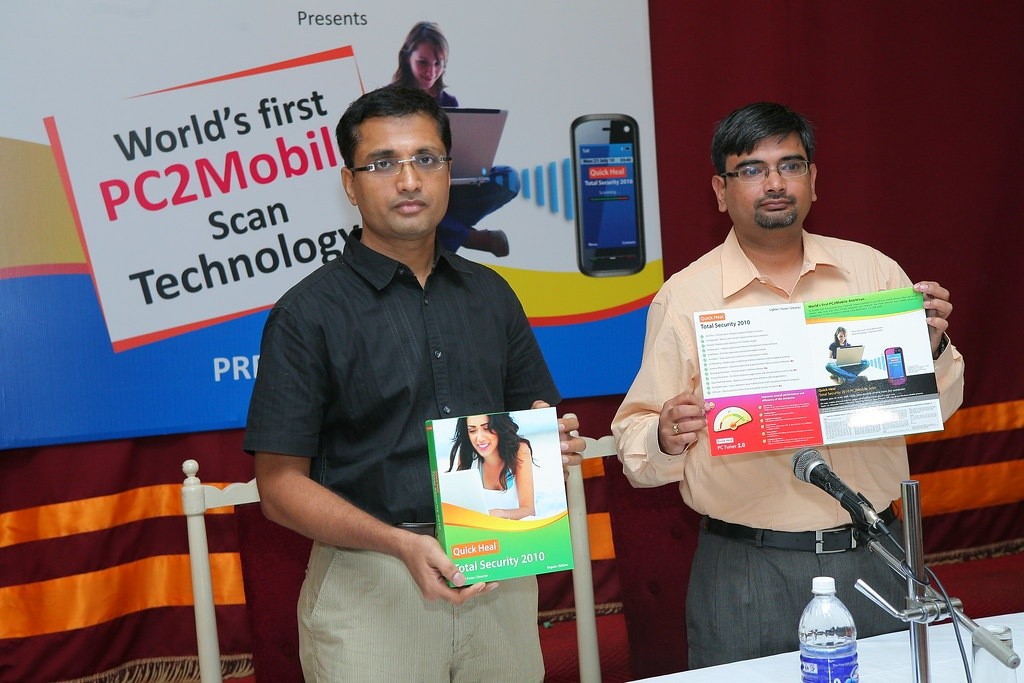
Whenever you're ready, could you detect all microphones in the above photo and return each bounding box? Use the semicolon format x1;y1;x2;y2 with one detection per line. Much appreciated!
790;446;890;537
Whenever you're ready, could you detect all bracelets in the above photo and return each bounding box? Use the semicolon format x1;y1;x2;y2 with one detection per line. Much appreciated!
939;337;947;355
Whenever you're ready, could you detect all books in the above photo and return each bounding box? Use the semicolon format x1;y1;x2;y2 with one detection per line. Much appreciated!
693;287;944;456
425;407;574;588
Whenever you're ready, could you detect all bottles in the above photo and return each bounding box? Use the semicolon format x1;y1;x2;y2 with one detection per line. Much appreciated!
798;577;860;683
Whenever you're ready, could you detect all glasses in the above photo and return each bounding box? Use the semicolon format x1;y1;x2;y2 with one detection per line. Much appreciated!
349;154;451;177
720;161;811;183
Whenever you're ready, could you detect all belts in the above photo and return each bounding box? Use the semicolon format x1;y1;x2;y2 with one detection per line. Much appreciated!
396;522;438;540
702;501;899;554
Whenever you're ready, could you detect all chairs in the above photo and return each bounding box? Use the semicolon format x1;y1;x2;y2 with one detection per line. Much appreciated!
563;414;706;683
181;457;314;683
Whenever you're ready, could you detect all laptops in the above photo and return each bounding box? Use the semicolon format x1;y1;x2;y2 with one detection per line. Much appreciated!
836;344;865;368
446;108;510;184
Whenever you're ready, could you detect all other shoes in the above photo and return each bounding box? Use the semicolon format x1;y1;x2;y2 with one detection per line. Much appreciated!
837;376;844;383
854;376;867;383
461;225;509;257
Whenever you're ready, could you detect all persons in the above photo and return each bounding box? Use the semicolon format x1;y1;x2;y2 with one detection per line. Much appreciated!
240;84;586;683
444;413;536;520
390;22;521;258
610;102;965;671
826;327;869;383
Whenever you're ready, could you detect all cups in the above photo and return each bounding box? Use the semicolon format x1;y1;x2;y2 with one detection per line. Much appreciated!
971;625;1018;683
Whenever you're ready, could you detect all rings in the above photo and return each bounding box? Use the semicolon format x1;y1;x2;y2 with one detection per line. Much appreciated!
673;424;679;435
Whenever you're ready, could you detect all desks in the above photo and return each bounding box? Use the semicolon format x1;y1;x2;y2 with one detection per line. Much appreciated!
625;613;1024;683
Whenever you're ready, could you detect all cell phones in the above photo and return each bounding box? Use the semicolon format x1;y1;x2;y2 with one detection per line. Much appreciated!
884;347;907;385
569;113;645;278
